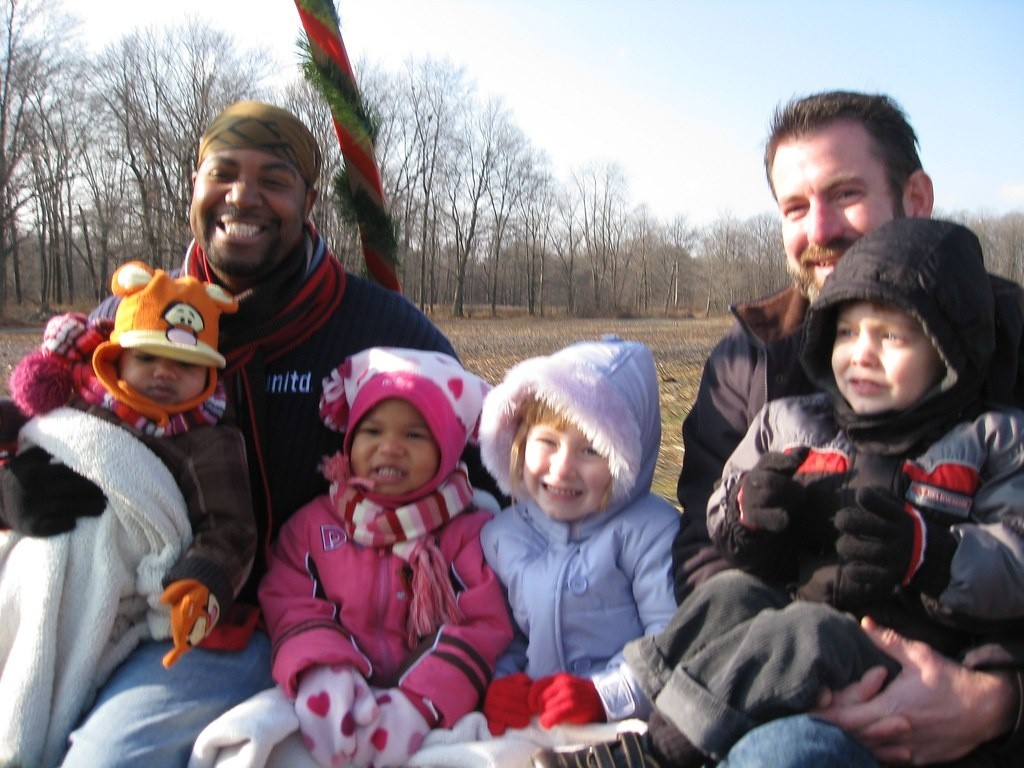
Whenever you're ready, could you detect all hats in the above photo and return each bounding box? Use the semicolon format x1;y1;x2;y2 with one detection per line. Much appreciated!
196;100;322;187
319;345;494;507
92;260;238;427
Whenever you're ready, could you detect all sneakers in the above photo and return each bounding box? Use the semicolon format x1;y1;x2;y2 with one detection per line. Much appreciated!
530;731;659;768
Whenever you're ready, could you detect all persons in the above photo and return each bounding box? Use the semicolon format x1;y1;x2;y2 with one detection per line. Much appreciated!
1;94;1024;768
479;341;684;733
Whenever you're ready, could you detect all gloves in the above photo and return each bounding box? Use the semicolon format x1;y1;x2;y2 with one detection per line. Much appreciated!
0;445;107;537
836;484;958;600
161;579;220;669
296;662;378;768
350;687;430;768
527;673;608;731
484;673;532;736
738;446;810;532
